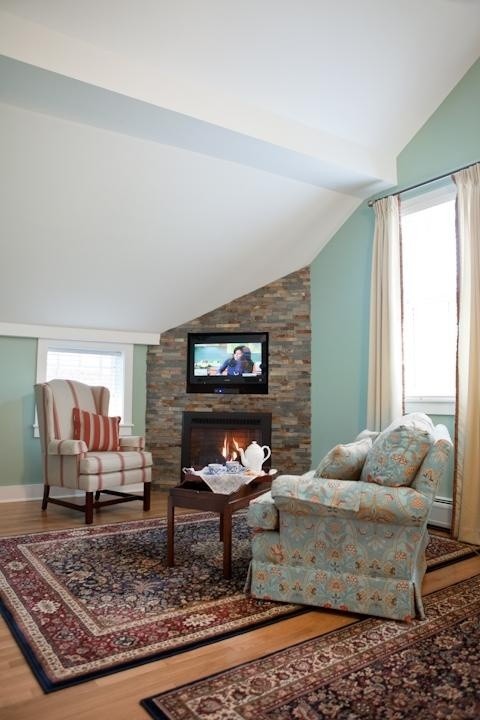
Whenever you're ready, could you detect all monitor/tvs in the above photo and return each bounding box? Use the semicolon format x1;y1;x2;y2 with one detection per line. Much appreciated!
186;332;269;394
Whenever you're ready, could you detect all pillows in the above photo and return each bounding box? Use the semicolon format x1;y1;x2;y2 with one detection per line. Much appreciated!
312;437;372;480
72;407;124;452
360;412;434;486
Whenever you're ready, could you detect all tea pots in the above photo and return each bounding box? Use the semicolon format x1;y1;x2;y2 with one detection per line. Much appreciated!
239;441;271;471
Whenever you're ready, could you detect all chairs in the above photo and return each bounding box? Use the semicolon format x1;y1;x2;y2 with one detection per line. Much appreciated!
242;423;454;623
35;379;153;524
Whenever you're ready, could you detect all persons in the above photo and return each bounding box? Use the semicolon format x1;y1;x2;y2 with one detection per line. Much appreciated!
214;345;253;376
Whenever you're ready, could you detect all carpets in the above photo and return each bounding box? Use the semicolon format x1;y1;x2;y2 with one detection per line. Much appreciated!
140;572;480;720
0;502;480;696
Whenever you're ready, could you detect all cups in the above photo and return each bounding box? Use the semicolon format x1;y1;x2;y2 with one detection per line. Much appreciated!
226;461;243;473
208;463;227;475
